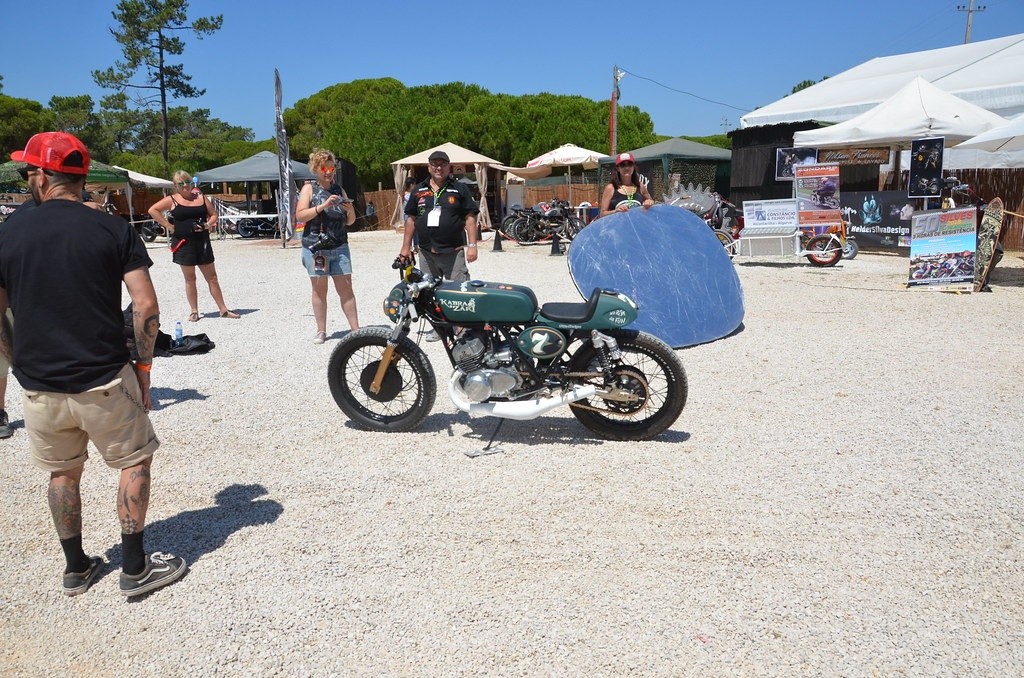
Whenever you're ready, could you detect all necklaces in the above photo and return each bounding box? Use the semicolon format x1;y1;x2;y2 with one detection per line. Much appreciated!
621;183;635;208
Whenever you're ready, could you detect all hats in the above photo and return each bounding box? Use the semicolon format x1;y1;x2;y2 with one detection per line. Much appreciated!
615;153;635;165
10;132;91;174
428;151;450;162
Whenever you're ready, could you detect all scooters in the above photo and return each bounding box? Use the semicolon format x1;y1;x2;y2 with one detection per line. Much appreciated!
683;194;737;260
840;208;859;260
810;189;840;209
140;205;174;243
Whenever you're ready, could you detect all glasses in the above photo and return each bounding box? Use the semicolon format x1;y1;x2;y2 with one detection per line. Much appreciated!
17;167;52;181
430;161;448;169
319;166;336;173
178;179;193;187
620;162;633;168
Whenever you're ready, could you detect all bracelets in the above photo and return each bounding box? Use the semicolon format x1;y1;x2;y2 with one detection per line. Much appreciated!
206;222;211;227
315;205;318;214
135;363;152;373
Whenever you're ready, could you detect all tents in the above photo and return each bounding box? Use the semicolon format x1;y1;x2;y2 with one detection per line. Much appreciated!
105;151;315;214
739;31;1024;170
793;76;1009;163
389;142;504;231
598;137;732;201
0;154;129;192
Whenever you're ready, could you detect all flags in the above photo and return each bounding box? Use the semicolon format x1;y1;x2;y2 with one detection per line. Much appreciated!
275;68;296;242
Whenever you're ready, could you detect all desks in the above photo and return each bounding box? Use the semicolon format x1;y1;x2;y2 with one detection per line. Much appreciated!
219;214;279;239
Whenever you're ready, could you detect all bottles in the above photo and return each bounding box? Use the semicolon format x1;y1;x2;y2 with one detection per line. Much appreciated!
175;322;183;347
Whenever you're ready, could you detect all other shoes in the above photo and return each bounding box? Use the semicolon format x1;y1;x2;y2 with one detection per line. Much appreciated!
313;331;327;344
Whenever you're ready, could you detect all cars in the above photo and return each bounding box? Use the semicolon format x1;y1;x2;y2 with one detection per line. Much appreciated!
665;191;745;241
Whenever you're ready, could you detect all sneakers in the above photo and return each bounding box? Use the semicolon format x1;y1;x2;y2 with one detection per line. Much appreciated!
62;556;104;595
0;411;14;438
119;551;187;596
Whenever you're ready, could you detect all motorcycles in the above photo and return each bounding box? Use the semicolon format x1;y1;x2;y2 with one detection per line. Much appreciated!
236;204;294;242
326;266;690;452
500;196;586;246
909;173;941;196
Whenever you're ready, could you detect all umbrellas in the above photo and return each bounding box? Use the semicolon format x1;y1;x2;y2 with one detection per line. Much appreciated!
948;115;1024;153
527;143;609;206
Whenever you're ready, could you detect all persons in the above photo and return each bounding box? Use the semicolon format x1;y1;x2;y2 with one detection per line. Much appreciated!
914;252;974;276
599;153;655;217
813;177;835;204
297;149;481;344
403;177;419;253
366;202;373;223
0;132;241;597
468;243;476;247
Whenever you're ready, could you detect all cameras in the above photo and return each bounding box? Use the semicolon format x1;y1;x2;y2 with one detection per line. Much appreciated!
392;251;416;270
333;196;355;206
